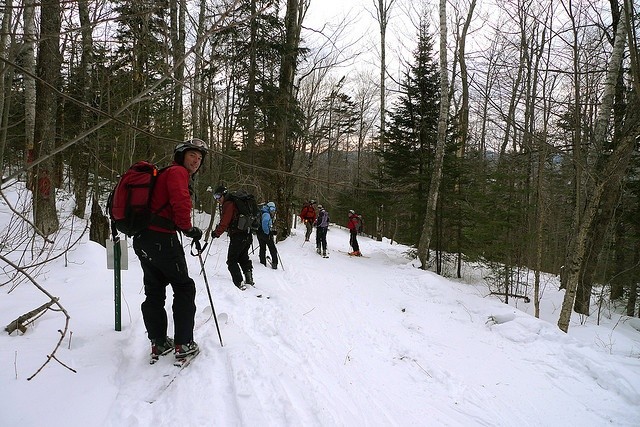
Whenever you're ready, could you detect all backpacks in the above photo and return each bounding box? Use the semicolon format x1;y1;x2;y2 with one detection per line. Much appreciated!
233;188;263;232
109;161;179;237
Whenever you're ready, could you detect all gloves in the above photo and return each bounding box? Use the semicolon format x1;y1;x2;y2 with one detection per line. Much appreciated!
185;227;203;240
211;231;218;239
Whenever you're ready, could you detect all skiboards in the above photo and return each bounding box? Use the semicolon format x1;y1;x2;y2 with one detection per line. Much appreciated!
266;256;274;266
338;249;371;258
141;306;228;403
316;249;330;258
245;281;271;298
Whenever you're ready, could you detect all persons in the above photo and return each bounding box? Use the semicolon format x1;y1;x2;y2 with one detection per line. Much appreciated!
256;200;279;270
211;186;255;291
347;209;362;257
299;200;316;244
312;202;331;257
127;137;209;359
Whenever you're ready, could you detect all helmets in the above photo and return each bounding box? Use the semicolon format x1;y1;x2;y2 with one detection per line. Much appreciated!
213;180;230;197
173;139;206;166
266;202;276;210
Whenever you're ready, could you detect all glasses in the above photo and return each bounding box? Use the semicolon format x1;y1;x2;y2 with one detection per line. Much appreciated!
212;193;222;200
269;206;276;211
190;137;208;149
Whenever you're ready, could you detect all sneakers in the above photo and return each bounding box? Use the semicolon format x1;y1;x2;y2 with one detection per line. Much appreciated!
246;278;255;286
239;280;246;290
175;340;198;357
150;337;175;355
